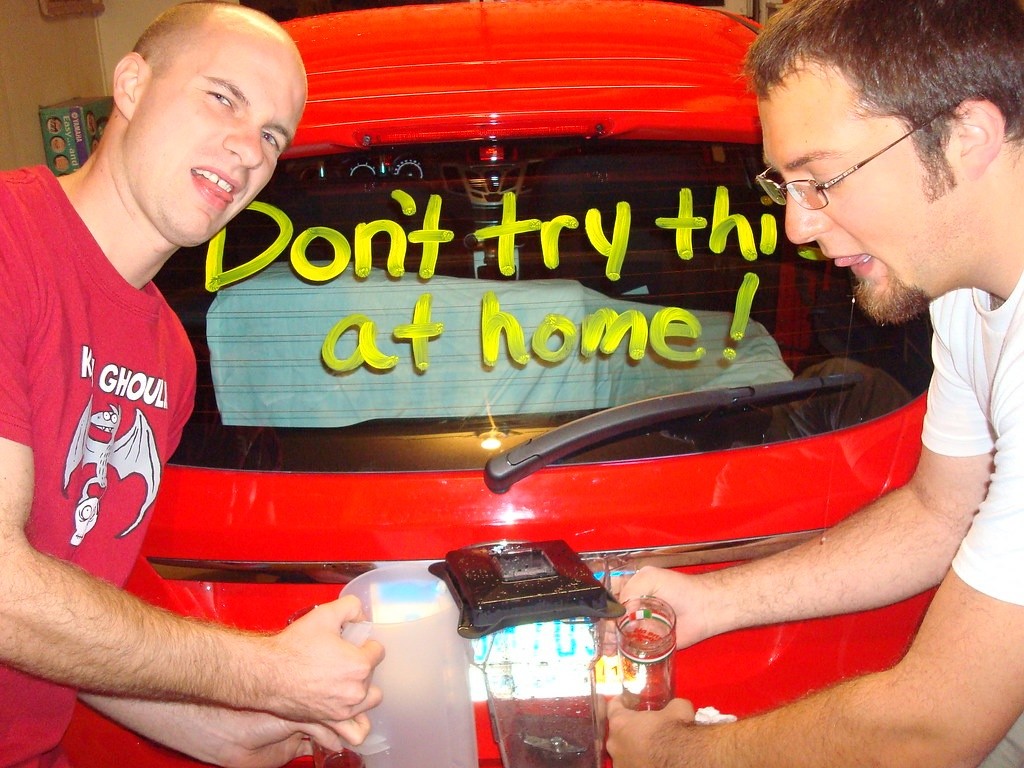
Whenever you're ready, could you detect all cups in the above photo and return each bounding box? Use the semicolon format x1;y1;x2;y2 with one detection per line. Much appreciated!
616;596;676;712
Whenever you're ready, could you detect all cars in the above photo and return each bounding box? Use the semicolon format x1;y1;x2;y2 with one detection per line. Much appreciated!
132;0;945;768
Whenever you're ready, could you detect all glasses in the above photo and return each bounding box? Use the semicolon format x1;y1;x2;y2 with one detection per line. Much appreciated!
755;90;993;210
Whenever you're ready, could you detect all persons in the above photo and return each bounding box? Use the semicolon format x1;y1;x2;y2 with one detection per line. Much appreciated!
600;0;1024;768
0;1;385;768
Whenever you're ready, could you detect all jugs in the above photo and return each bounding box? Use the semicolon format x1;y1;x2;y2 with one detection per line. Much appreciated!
339;566;479;768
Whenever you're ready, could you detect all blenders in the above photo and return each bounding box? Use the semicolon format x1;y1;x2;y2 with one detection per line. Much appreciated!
428;538;624;768
